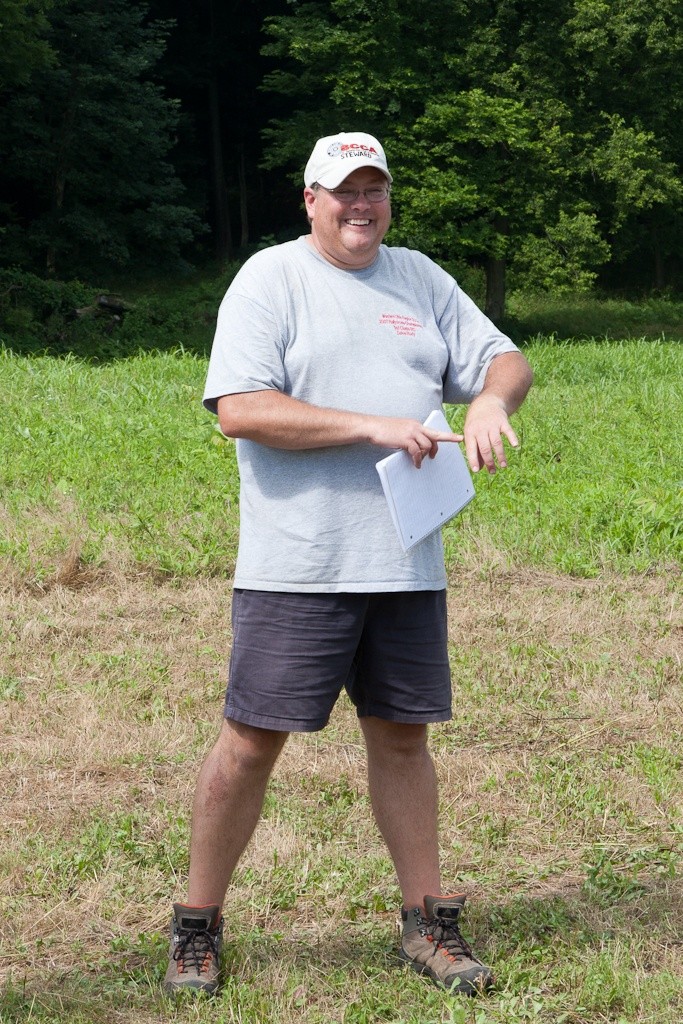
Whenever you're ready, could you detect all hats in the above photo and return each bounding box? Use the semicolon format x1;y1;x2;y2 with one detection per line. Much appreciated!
304;132;394;189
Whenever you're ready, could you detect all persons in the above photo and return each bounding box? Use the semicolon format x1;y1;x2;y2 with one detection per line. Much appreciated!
163;133;534;1001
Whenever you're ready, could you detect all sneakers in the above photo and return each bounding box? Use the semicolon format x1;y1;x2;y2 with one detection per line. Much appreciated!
398;893;493;997
164;902;224;1003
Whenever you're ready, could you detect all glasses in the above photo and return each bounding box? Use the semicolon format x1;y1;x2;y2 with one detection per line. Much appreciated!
329;186;392;203
